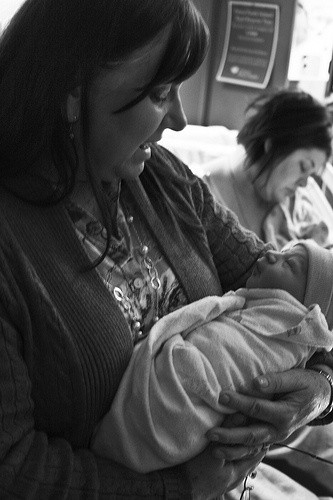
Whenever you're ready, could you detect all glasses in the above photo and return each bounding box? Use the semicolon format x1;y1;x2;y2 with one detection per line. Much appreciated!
240;443;333;500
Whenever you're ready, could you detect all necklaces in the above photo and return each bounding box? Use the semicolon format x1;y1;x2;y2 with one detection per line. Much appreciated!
96;196;161;339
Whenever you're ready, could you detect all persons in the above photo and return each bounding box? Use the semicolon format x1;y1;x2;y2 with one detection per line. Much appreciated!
89;239;333;472
0;0;333;500
200;87;333;250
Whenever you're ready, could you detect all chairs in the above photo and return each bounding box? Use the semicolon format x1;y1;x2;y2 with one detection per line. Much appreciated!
156;124;333;244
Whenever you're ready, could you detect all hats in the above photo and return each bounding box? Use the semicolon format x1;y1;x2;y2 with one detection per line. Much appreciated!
293;239;333;330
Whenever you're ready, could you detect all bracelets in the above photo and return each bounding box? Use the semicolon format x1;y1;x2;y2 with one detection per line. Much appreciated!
308;366;333;420
